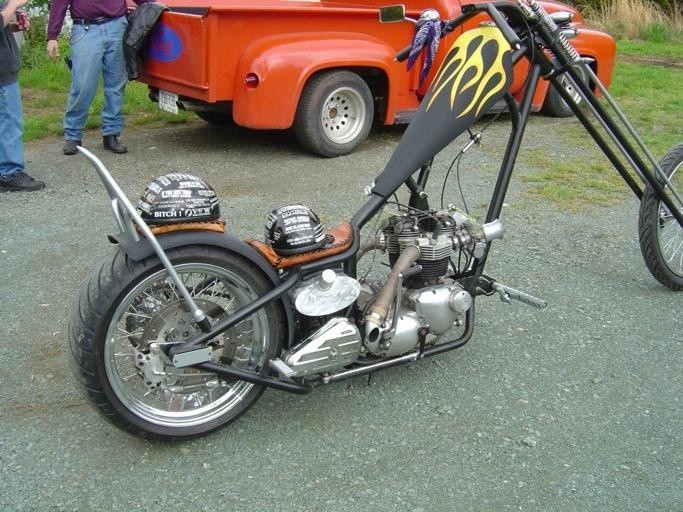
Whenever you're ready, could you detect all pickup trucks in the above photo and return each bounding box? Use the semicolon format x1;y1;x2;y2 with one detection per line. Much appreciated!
127;1;622;152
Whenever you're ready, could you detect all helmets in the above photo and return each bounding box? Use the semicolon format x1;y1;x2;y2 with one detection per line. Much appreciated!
133;173;220;224
265;204;327;254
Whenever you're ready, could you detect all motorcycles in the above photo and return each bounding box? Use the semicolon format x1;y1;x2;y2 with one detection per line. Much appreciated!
57;1;682;450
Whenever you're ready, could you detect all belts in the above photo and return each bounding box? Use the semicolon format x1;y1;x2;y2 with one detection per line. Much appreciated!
72;16;117;25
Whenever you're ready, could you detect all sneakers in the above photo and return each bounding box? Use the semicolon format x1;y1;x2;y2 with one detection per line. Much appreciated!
1;168;45;192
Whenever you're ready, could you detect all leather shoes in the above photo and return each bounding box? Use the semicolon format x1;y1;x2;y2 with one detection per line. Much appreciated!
62;140;81;155
103;135;126;153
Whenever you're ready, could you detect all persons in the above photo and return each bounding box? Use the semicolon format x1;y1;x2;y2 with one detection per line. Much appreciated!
46;0;156;155
0;0;45;193
